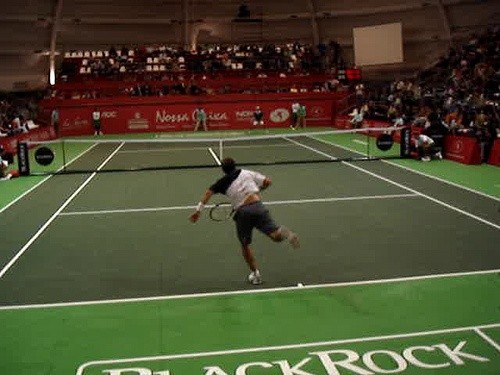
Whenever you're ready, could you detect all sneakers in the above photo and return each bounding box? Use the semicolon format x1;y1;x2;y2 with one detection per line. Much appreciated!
249;271;262;285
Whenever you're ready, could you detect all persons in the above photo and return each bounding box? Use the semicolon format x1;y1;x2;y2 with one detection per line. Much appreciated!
0;81;40;183
346;81;376;128
51;107;60;135
296;105;307;131
290;99;300;130
192;107;207;131
59;37;343;91
377;32;499;133
92;107;104;136
413;132;443;162
189;157;300;285
248;105;269;135
